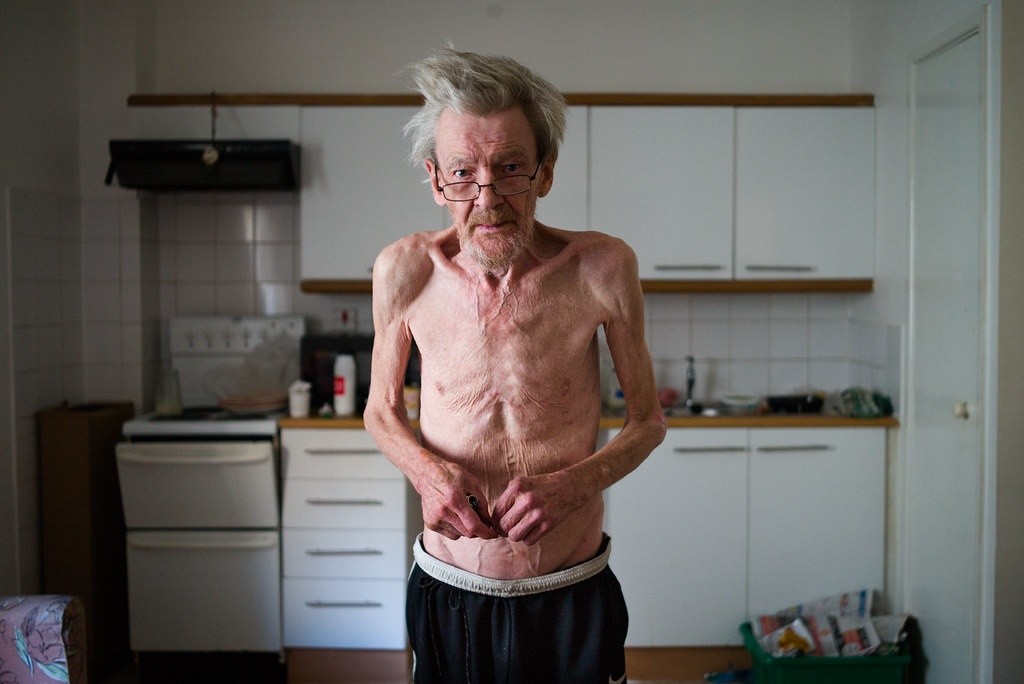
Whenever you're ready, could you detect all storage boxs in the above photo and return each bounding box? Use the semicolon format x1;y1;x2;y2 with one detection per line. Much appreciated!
738;622;910;683
37;400;135;684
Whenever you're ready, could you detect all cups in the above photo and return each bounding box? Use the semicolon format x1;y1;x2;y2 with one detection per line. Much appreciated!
290;393;310;418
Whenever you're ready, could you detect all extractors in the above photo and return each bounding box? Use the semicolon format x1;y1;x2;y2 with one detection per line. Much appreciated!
103;135;303;197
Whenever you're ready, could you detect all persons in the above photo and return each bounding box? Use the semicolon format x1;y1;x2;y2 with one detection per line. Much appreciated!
362;49;668;684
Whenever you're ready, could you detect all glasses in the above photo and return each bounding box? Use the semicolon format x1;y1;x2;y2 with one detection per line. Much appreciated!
434;151;546;202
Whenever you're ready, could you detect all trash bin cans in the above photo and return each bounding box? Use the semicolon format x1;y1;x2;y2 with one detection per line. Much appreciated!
737;622;913;684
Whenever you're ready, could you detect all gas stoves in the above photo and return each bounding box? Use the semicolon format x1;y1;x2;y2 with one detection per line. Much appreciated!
117;402;281;436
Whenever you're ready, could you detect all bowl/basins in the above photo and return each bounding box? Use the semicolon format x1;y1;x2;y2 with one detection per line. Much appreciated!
721;393;760;417
764;394;825;416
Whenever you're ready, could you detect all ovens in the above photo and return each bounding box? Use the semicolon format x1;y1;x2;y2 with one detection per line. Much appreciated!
114;440;284;653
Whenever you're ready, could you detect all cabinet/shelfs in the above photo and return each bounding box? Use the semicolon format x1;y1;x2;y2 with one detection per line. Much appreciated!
587;93;878;293
602;426;886;650
278;429;422;649
116;439;282;652
298;92;587;294
125;93;303;144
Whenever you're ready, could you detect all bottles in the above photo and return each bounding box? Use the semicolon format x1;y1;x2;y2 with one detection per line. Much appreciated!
607;369;626;407
333;344;357;417
